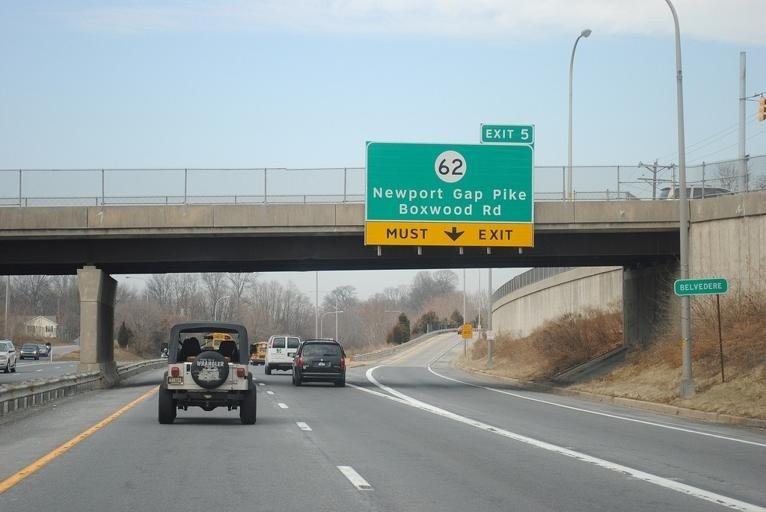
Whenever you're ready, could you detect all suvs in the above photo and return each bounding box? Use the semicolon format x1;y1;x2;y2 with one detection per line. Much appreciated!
159;322;256;425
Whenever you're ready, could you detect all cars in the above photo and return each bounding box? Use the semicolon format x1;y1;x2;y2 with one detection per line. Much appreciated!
0;340;17;373
292;338;346;386
659;186;736;200
458;326;463;334
251;342;268;366
20;343;51;360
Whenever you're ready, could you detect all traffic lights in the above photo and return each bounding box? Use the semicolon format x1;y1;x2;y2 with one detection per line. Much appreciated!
758;98;766;121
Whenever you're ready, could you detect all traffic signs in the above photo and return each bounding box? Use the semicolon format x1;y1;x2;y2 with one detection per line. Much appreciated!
365;141;534;246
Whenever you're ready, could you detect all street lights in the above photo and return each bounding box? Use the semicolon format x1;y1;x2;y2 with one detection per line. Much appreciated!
214;295;230;321
566;29;592;200
309;289;343;342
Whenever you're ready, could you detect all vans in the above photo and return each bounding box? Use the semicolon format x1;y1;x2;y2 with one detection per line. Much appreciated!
265;335;300;375
